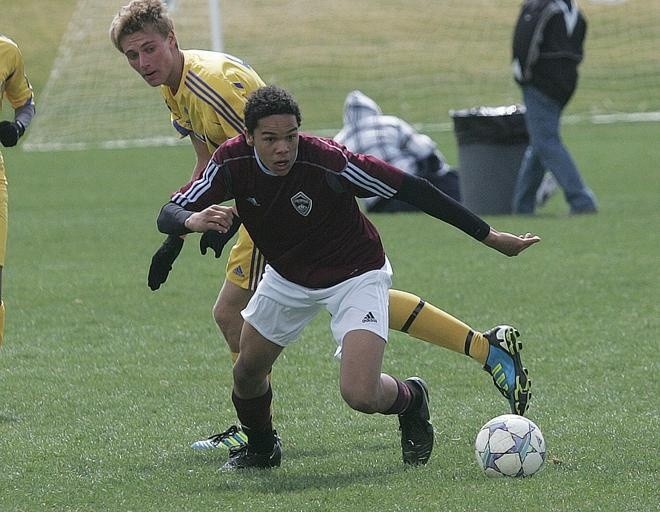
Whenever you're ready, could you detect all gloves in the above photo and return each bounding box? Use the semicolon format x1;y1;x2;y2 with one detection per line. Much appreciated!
146;234;185;292
198;209;244;260
0;120;26;147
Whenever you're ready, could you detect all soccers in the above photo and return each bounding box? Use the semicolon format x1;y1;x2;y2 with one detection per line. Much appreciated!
476;414;546;476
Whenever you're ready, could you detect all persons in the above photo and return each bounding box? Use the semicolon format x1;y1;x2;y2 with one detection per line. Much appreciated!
508;1;601;214
333;90;461;219
156;84;542;472
104;0;533;450
0;36;37;343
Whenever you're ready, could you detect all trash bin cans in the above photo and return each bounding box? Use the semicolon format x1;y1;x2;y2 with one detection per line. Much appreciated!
451;110;530;215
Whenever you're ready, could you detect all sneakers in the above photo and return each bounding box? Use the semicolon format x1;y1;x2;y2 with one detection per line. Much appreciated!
483;323;534;418
215;438;283;474
189;424;279;453
398;374;434;465
